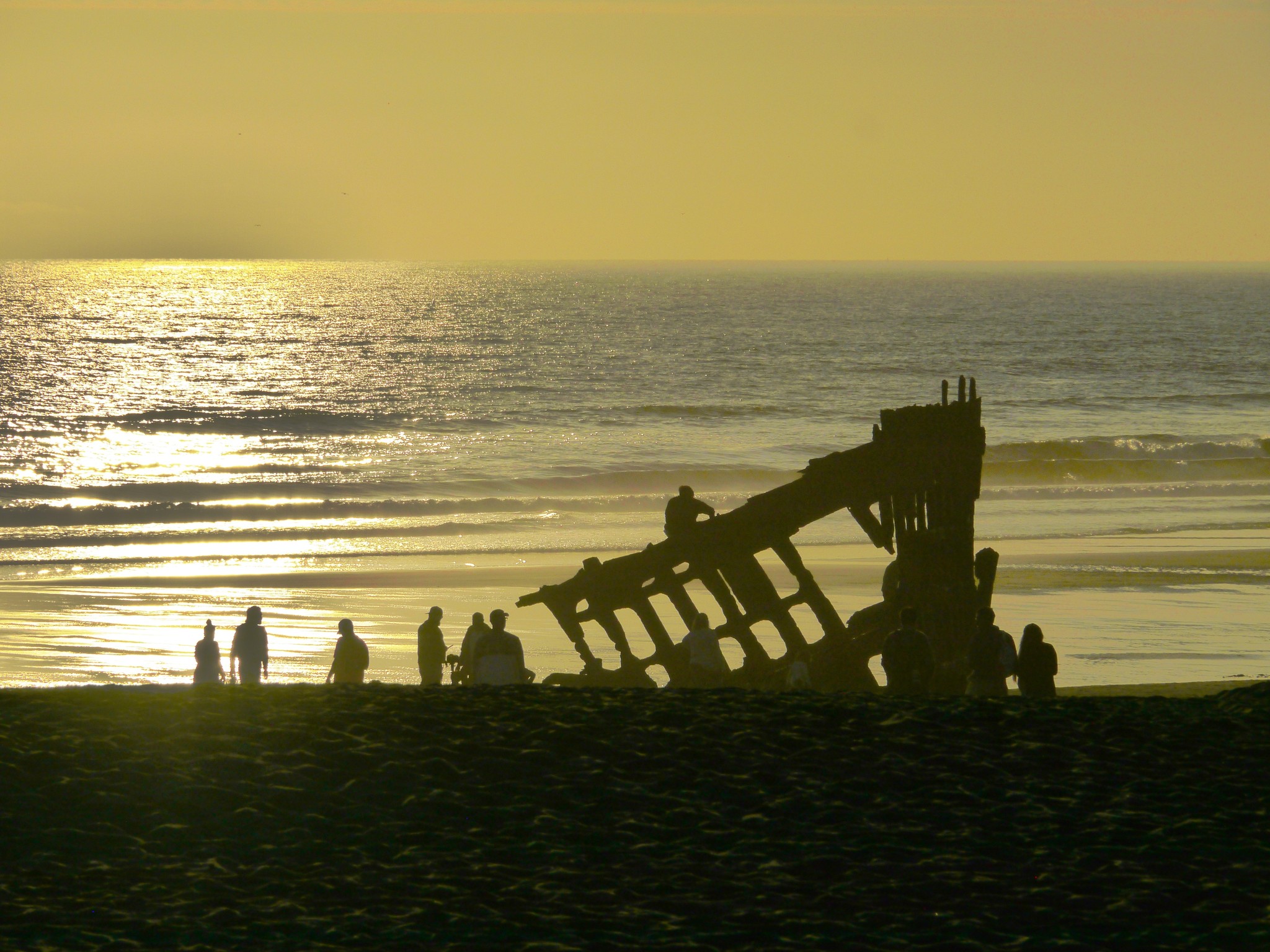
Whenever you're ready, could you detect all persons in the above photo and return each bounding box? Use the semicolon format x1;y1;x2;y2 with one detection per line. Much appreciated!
455;609;536;685
418;606;448;685
325;619;369;683
193;619;226;682
881;606;936;686
663;486;715;537
682;613;720;688
965;607;1018;696
229;606;268;683
1013;623;1058;697
